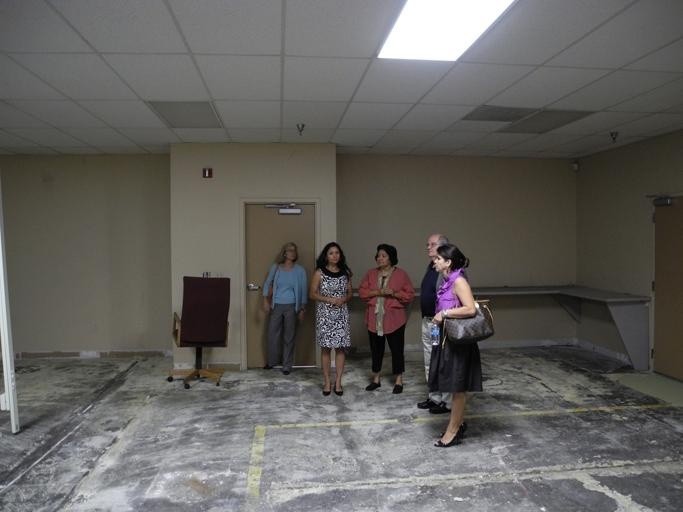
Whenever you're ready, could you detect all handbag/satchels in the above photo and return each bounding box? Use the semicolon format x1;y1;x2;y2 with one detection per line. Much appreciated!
268;284;273;299
443;301;495;346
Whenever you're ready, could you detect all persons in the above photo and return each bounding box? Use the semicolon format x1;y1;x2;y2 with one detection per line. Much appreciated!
261;241;309;375
358;243;416;394
426;242;483;448
309;241;354;396
417;233;452;415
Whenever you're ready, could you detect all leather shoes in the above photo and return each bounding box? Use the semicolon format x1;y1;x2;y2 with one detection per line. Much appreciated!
263;364;273;369
392;383;404;394
283;370;289;375
365;381;381;391
417;397;439;408
334;382;344;396
323;382;331;395
430;401;451;414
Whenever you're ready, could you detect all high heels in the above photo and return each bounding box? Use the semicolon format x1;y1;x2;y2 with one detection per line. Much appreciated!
434;429;458;447
436;421;466;439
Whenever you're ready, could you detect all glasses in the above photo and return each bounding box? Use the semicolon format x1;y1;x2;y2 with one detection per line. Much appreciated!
286;249;297;253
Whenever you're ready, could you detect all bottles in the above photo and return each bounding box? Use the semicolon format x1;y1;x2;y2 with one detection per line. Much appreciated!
430;323;439;346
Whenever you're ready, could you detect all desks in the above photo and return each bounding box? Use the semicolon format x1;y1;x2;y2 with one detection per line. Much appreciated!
348;283;655;373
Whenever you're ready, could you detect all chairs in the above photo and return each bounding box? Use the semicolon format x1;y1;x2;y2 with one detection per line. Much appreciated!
166;274;232;391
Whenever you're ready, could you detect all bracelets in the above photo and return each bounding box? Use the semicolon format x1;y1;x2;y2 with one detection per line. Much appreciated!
442;309;449;319
377;288;382;295
